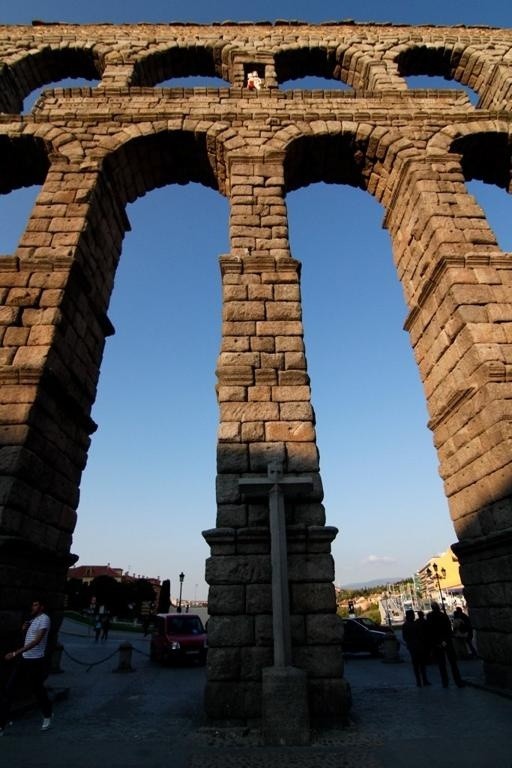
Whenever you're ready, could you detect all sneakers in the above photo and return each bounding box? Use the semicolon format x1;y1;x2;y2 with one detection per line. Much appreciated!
457;681;467;688
40;712;54;731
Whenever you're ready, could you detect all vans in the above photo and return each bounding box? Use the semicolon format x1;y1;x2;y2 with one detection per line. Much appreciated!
150;612;208;667
342;619;400;657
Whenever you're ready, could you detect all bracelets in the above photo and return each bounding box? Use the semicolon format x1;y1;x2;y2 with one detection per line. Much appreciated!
13;651;16;658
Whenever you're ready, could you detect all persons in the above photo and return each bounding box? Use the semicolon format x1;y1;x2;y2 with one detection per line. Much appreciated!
402;601;475;688
128;598;155;637
85;596;113;646
4;600;55;732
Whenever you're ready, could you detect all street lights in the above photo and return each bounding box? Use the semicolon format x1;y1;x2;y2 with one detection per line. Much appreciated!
427;563;447;615
179;572;184;610
